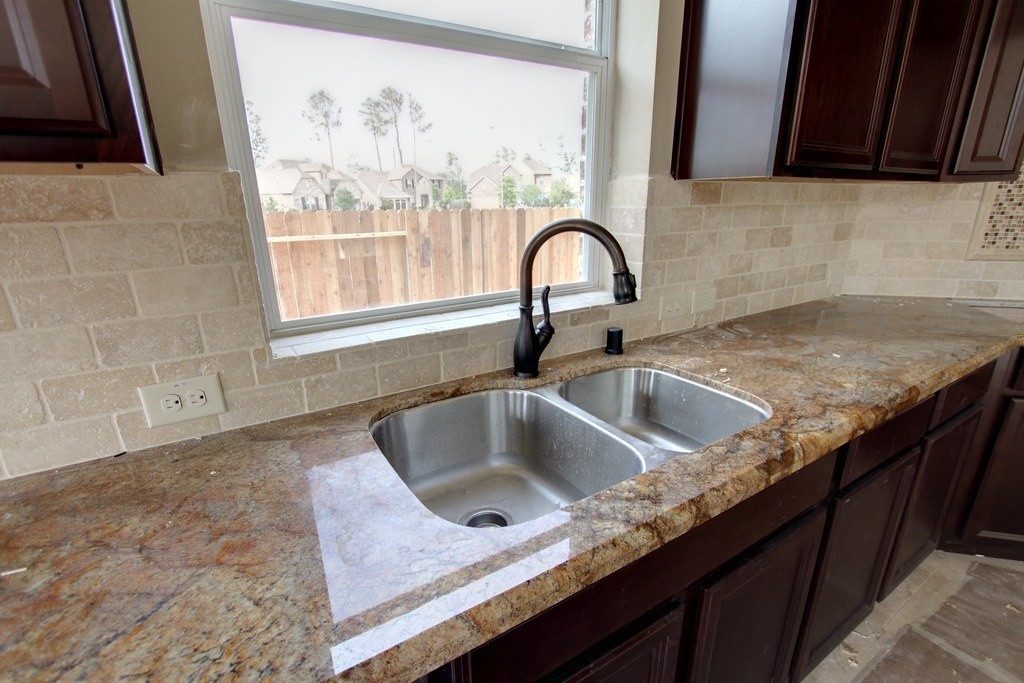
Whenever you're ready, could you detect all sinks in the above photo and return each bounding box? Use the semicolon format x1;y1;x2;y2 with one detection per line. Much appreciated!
367;386;646;529
556;363;774;454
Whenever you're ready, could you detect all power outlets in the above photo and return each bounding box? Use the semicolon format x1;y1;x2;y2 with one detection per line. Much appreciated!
136;374;225;426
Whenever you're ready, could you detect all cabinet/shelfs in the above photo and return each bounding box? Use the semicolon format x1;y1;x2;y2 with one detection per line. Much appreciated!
418;450;839;683
936;346;1024;561
671;0;1024;184
790;361;998;683
0;0;164;179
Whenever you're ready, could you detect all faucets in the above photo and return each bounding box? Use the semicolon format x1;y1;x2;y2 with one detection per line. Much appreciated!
512;217;638;395
